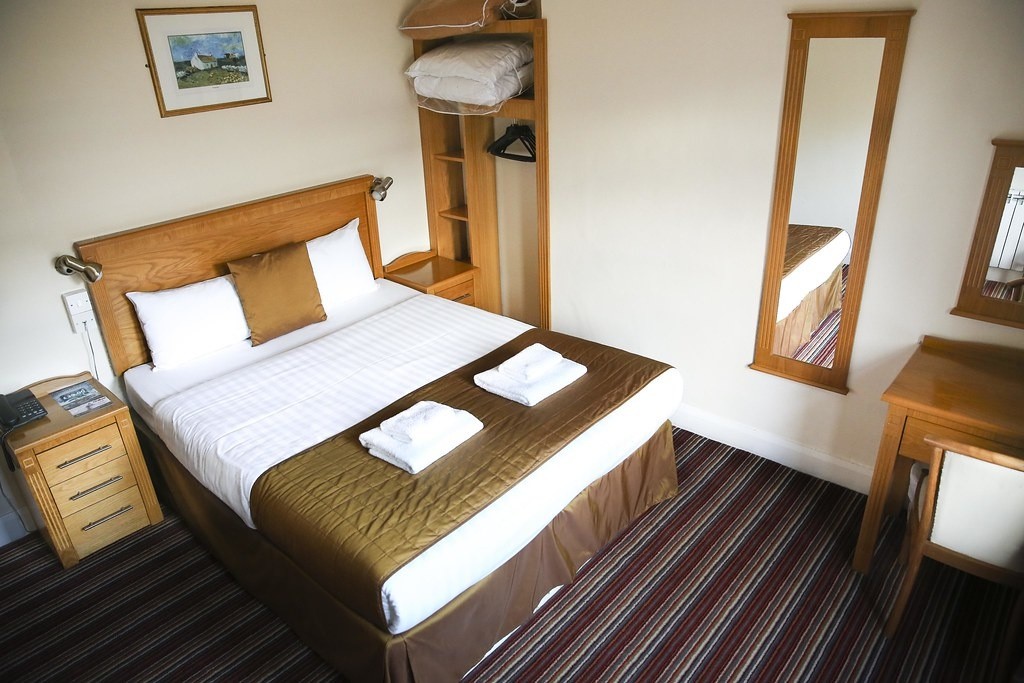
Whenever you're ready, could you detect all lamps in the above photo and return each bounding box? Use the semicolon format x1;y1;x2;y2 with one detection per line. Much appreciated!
56;254;103;284
371;176;394;201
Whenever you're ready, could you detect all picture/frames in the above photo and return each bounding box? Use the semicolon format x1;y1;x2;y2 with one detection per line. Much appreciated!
135;5;273;119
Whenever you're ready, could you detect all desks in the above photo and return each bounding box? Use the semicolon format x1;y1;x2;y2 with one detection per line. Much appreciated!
852;334;1024;572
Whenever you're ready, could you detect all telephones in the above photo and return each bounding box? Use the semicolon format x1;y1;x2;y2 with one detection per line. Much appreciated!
0;388;48;432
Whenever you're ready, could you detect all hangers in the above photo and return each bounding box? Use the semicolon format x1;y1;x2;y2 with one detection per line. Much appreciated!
486;119;536;164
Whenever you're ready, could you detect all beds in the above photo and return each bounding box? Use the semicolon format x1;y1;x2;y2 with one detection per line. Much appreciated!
73;171;683;683
775;221;851;360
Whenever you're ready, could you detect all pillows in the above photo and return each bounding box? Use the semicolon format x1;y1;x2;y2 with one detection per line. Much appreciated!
226;240;327;346
126;274;253;374
404;40;534;106
303;217;378;316
398;0;538;39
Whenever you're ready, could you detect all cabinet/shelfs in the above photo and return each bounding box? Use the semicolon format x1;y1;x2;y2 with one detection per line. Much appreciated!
414;16;549;331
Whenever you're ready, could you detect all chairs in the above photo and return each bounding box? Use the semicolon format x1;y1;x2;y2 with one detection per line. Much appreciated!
882;434;1024;638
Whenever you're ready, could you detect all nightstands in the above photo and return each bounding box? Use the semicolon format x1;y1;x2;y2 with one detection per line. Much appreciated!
382;250;481;309
4;371;165;571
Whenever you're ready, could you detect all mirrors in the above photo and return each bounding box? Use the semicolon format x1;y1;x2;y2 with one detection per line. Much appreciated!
949;139;1023;329
746;10;913;394
